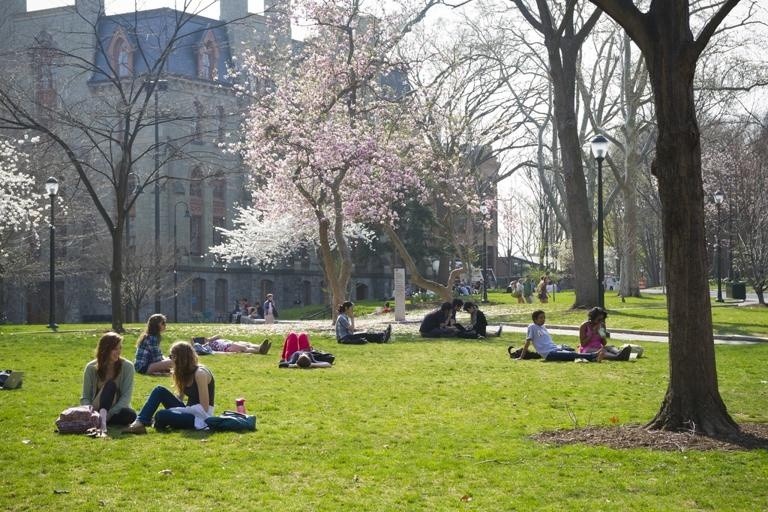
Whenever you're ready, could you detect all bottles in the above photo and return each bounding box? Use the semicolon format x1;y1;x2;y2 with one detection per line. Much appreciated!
236;399;244;415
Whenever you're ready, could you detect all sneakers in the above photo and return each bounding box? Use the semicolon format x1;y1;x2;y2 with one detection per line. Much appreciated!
258;338;272;354
383;325;393;342
596;346;605;363
496;323;503;337
618;344;630;361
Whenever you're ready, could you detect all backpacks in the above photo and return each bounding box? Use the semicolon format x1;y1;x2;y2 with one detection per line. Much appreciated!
55;404;101;436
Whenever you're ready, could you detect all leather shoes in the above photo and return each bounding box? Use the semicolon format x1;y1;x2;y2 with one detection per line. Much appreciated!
121;424;147;435
95;427;108;438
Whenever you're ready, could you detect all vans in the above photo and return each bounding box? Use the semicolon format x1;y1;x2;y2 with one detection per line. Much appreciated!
605;274;620;292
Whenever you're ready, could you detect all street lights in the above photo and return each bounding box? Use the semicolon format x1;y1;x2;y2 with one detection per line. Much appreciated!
144;75;171;320
172;198;193;322
590;132;608;312
477;201;492;303
713;187;725;304
122;173;144;322
44;175;61;328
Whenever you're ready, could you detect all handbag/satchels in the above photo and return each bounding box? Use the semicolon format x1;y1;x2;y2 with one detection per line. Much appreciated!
0;370;24;389
620;342;644;357
506;344;542;360
192;342;213;355
206;409;257;432
310;349;336;364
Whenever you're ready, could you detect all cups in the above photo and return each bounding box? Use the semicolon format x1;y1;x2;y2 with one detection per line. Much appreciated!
598;327;607;340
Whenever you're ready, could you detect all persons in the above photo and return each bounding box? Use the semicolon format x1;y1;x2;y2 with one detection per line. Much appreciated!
334;301;392;343
580;306;631;361
410;278;501;340
513;310;606;363
511;274;551;303
81;293;336;433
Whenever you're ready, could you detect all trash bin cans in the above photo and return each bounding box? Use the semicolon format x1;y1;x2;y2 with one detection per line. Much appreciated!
725;280;747;301
